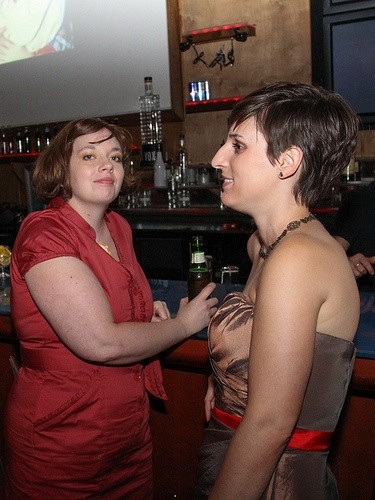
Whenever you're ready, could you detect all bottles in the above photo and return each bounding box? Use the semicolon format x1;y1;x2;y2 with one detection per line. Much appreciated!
0;126;59;154
152;135;189;187
188;235;212;302
138;77;162;144
342;156;362;181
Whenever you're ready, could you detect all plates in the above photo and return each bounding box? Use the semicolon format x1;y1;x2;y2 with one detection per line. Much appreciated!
346;177;374;186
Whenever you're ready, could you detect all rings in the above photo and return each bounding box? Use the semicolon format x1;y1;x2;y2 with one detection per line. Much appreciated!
356;264;359;267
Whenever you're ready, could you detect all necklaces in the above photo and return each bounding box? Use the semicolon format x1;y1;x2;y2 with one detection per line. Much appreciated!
258;216;316;258
97;241;111;255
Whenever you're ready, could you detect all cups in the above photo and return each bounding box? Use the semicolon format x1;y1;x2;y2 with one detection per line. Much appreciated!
220;266;240;294
118;165;212;208
0;253;12;296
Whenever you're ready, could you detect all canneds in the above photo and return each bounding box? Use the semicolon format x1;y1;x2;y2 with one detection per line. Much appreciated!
197;165;210;184
187;166;197;185
197;80;209;101
188;81;199;102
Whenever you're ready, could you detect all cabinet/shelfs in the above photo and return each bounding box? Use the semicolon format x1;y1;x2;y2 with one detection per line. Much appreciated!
1;95;375;283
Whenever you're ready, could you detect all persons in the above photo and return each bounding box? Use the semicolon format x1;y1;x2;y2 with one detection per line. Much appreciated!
192;82;359;500
5;116;219;500
334;180;375;293
